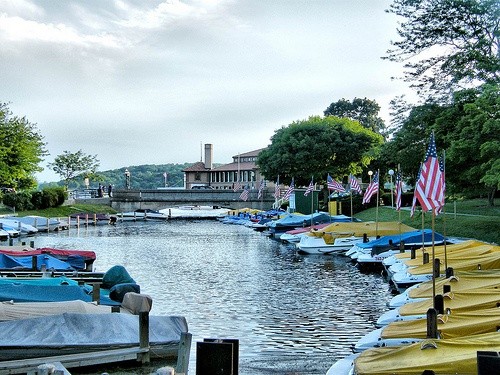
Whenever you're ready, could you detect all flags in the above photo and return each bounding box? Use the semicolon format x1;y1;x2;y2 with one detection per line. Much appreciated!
350;174;363;195
410;133;445;219
274;178;281;198
257;180;264;198
304;180;314;197
362;172;378;204
233;178;241;192
396;169;402;211
284;178;295;200
240;183;250;201
327;176;346;193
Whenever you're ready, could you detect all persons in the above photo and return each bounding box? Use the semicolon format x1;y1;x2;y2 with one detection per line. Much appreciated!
99;183;112;197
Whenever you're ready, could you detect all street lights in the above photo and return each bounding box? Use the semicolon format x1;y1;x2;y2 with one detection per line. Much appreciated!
388;169;394;209
368;170;373;186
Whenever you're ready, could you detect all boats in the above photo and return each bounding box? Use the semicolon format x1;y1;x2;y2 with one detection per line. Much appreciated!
215;206;500;375
0;208;189;375
